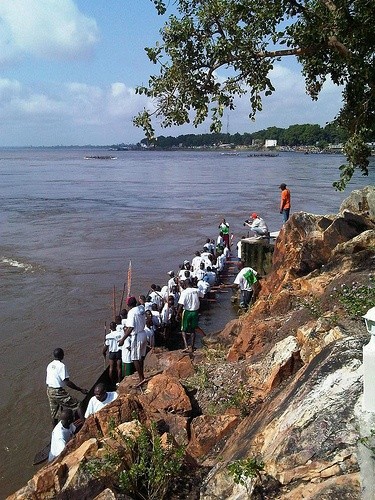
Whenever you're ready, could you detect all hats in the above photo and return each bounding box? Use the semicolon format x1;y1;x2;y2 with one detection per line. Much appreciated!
252;213;257;218
127;296;135;305
168;271;172;275
279;183;287;188
184;260;189;264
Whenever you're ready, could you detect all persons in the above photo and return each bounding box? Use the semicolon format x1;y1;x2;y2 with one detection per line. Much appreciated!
244;213;269;239
279;183;291;224
49;409;82;464
45;348;88;429
219;261;261;312
103;218;230;387
85;384;119;419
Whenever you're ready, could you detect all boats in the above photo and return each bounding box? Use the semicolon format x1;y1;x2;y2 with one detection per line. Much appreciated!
30;363;127;466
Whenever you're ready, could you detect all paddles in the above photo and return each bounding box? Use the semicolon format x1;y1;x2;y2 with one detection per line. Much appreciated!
118;283;125;315
104;320;106;371
112;284;117;322
125;259;132;308
